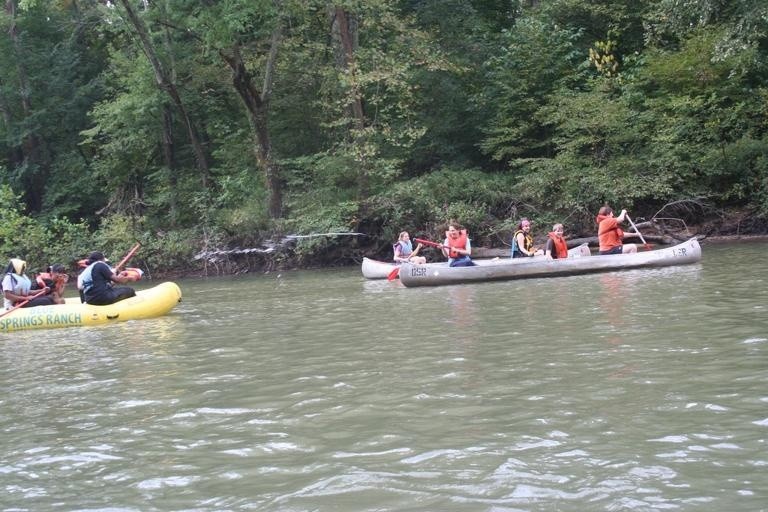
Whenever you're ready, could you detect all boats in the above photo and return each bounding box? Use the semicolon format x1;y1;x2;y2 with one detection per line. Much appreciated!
397;235;706;292
0;281;185;334
354;240;592;279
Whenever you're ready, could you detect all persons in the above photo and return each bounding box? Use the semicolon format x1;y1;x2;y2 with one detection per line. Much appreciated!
596;205;637;255
546;223;568;261
0;251;135;307
437;224;471;267
393;231;426;264
511;220;537;257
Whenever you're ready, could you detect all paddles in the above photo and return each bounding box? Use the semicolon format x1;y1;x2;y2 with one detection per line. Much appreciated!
626;213;653;251
412;238;467;258
386;244;423;281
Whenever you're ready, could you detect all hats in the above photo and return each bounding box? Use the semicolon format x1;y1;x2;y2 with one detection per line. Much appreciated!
89;252;106;264
51;263;67;274
